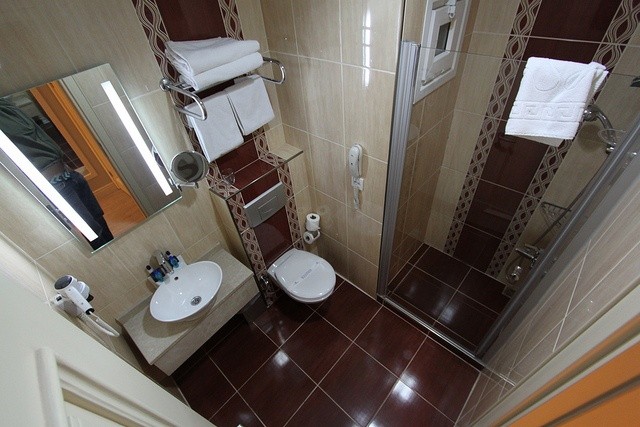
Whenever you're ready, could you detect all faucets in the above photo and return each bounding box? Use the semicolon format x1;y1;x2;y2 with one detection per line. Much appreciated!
151;250;178;276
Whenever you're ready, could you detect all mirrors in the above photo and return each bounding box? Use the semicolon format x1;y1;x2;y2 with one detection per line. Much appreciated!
1;64;183;256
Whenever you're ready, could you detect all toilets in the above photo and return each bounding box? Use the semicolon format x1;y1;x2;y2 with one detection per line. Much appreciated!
261;247;340;322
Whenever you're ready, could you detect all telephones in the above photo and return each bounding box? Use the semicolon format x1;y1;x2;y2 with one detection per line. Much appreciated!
348;145;363;210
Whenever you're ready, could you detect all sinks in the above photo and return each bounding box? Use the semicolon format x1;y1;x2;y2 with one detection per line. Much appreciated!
145;255;224;324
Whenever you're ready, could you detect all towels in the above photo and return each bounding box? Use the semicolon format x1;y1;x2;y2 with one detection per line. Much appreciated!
226;75;274;134
181;94;246;162
158;39;273;84
504;56;609;148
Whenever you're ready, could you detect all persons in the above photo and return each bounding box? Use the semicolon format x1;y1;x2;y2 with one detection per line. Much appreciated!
0;96;115;252
